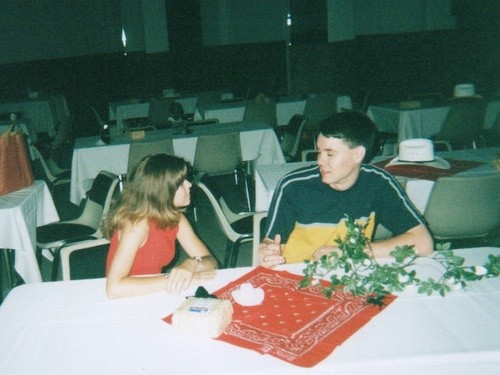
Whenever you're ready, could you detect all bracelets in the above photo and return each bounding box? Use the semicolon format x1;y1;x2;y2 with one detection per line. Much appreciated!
193;256;202;269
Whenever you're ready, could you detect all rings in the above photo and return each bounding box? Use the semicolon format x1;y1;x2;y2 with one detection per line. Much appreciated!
263;257;268;262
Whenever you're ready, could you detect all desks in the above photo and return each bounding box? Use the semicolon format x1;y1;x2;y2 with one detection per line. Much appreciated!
195;94;352;129
110;91;233;129
0;121;37;161
366;97;500;150
0;180;60;292
0;247;500;375
0;92;70;137
70;121;287;221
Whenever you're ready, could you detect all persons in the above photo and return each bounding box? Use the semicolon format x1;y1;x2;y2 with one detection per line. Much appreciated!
256;110;434;269
432;83;488;152
99;152;219;301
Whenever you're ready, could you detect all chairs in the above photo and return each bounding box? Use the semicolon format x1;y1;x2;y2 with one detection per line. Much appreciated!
35;95;500;302
